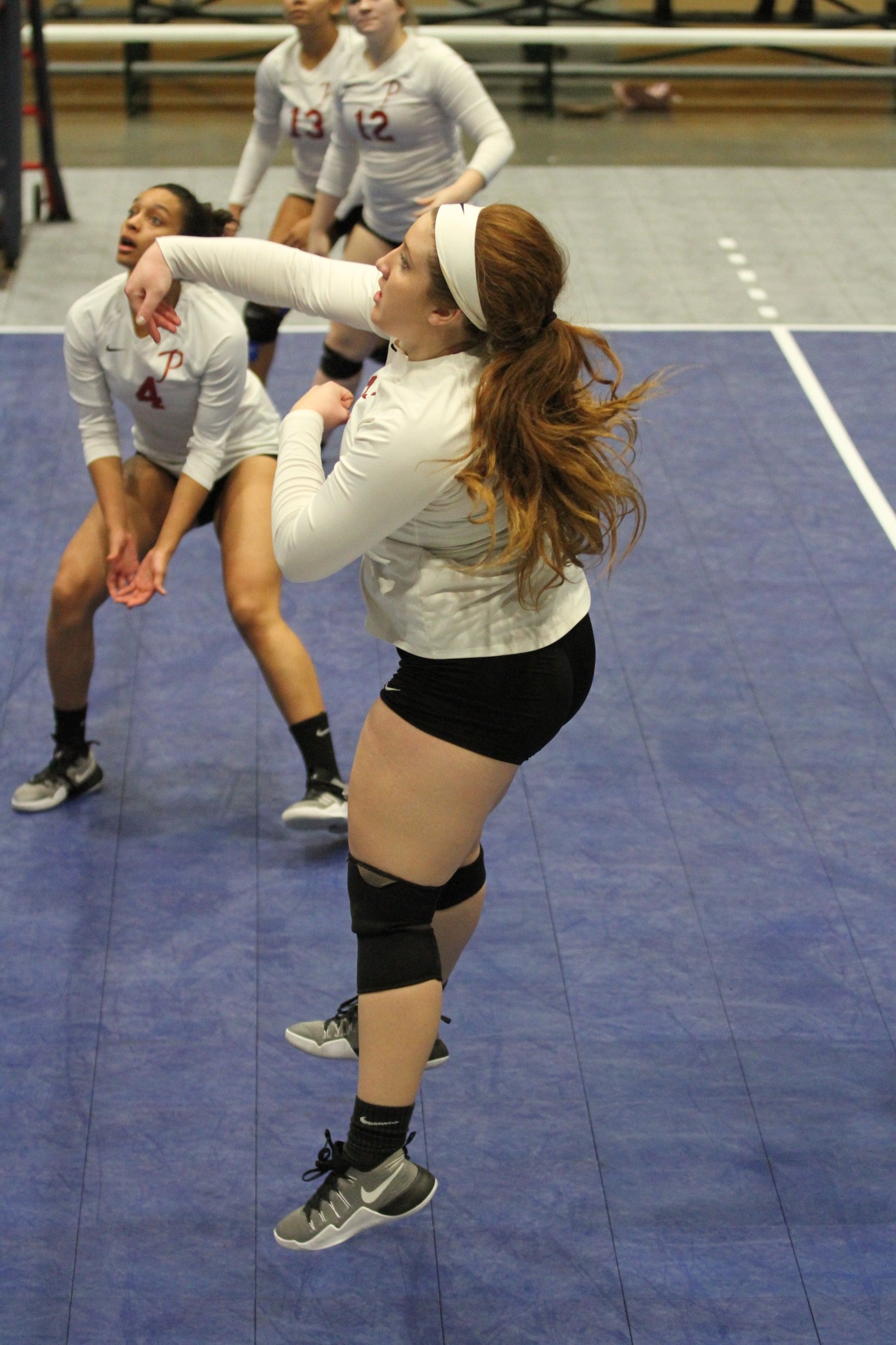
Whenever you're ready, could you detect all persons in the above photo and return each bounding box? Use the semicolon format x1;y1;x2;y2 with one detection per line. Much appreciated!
307;0;519;451
124;203;673;1251
220;0;386;384
9;184;349;831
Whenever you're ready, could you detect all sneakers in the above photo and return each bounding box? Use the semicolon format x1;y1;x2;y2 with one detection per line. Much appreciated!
281;769;352;834
271;1128;440;1252
10;740;107;814
284;991;455;1068
